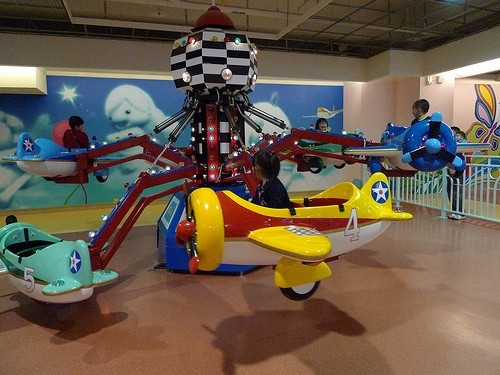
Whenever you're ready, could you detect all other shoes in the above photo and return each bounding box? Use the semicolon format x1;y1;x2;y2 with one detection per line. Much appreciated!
447;213;466;220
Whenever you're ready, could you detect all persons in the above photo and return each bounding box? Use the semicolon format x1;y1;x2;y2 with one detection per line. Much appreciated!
411;98;431;127
249;149;294;215
63;116;89;149
315;118;328;131
446;126;473;220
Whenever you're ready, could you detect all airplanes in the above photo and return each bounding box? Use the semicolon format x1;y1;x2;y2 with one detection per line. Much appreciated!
265;128;367;175
1;132;110;183
0;215;120;304
175;173;415;302
343;111;493;179
